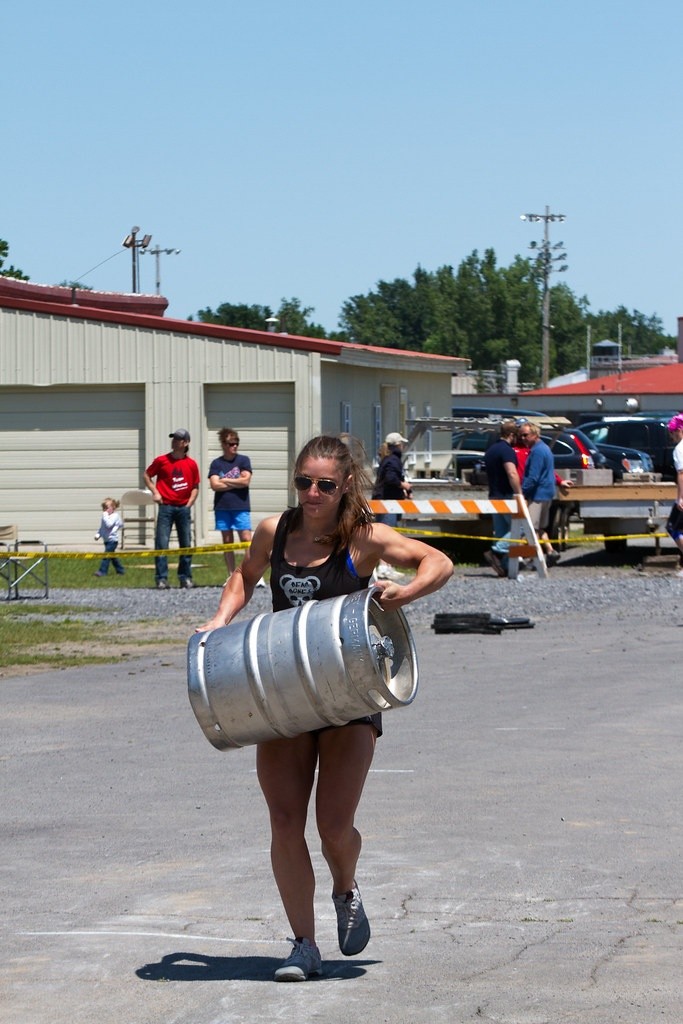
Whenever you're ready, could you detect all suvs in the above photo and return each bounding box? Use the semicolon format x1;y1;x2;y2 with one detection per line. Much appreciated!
451;403;683;480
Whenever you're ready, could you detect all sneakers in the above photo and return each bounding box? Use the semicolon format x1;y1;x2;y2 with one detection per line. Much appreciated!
274;936;321;981
332;879;370;956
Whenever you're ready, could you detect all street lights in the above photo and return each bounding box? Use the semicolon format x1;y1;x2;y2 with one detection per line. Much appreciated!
519;203;568;389
140;243;181;296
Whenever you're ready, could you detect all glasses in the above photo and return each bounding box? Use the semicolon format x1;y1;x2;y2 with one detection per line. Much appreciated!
519;433;532;436
224;442;238;447
292;476;346;497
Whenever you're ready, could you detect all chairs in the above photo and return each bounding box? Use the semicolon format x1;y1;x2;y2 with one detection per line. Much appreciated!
119;488;197;556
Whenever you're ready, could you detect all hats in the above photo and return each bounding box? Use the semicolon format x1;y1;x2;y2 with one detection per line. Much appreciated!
168;429;189;441
516;418;528;427
386;432;409;446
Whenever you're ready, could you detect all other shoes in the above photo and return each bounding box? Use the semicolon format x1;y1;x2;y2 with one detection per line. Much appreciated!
156;581;166;589
94;571;103;577
180;579;193;588
485;550;509;576
546;550;559;568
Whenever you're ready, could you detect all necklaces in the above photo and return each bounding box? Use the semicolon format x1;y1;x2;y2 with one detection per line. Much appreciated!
306;527;335;541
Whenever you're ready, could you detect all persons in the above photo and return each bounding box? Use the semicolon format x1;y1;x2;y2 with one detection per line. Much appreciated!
664;414;683;550
93;498;125;577
371;432;411;580
143;428;200;589
205;432;266;588
190;436;453;976
483;422;575;575
